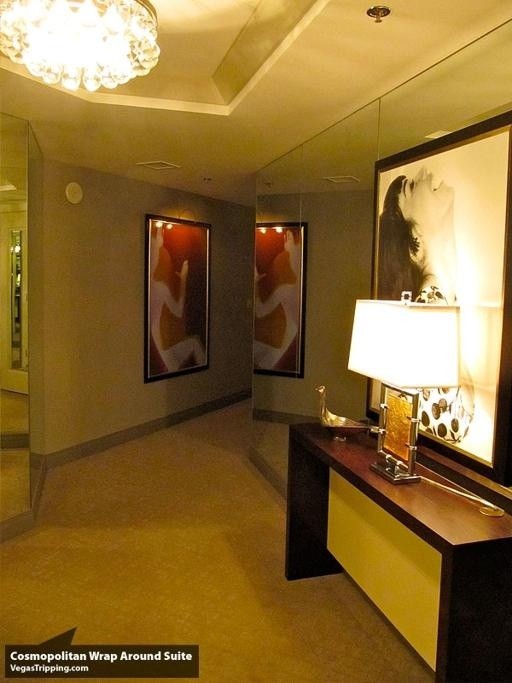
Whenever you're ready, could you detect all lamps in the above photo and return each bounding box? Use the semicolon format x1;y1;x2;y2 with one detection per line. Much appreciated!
2;0;163;97
346;297;462;483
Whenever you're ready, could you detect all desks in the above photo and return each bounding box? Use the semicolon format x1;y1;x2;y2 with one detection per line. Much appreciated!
283;422;512;682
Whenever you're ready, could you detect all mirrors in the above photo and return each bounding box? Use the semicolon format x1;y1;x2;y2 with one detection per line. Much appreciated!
9;227;23;373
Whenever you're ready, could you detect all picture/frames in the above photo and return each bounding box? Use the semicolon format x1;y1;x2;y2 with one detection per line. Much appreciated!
254;221;309;379
143;213;213;383
364;110;511;490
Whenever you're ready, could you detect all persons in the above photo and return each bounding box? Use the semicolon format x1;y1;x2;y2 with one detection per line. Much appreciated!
378;169;474;440
